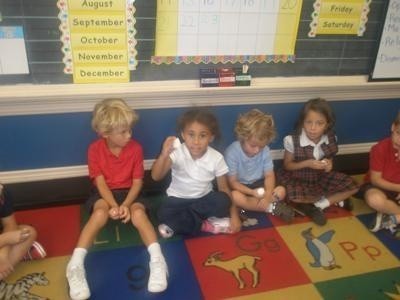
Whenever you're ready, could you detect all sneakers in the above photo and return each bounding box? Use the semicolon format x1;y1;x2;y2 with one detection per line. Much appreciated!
271;200;295;223
19;242;47;262
158;223;174;239
145;254;171;293
205;215;238;235
332;197;356;211
303;202;329;228
368;209;395;234
65;260;92;300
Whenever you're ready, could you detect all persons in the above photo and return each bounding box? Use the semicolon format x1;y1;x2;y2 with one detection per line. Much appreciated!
152;107;242;239
0;183;45;282
279;98;360;225
66;98;170;300
363;112;400;232
223;109;295;223
